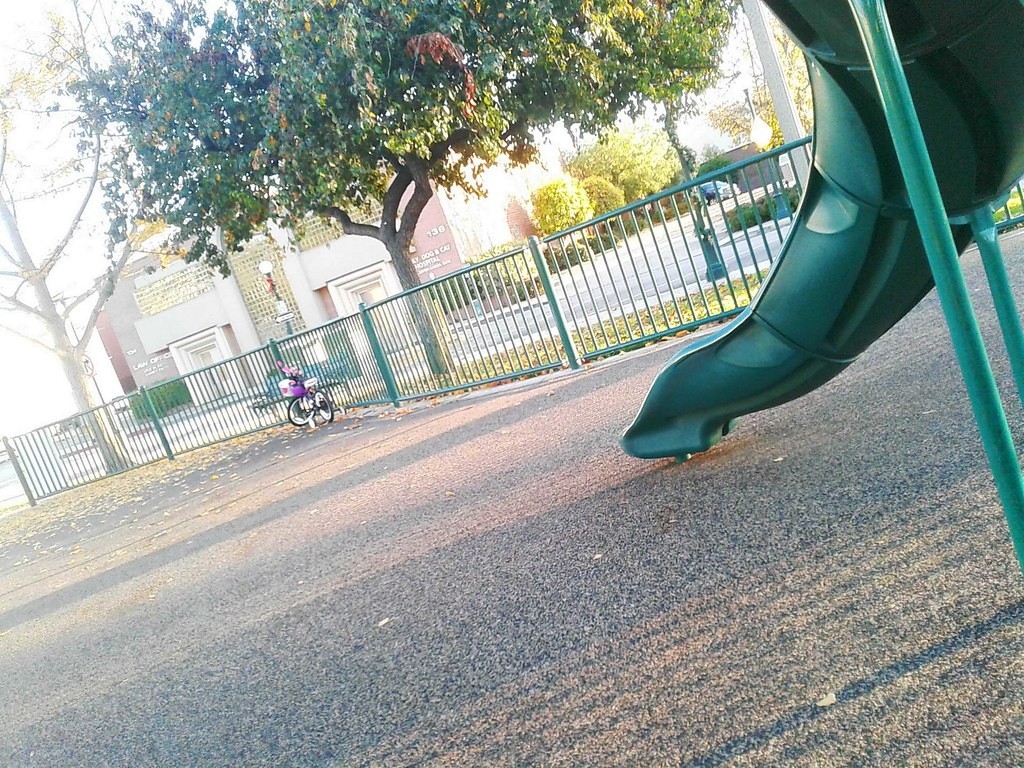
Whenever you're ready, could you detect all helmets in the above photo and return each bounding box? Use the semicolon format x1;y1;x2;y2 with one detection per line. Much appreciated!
289;382;308;398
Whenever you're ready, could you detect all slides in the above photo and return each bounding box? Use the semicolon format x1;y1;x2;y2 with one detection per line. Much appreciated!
620;0;1024;465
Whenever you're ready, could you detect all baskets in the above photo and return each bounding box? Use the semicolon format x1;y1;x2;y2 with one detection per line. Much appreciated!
278;379;295;397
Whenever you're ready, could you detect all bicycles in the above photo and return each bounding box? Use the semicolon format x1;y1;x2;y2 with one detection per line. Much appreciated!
277;361;335;427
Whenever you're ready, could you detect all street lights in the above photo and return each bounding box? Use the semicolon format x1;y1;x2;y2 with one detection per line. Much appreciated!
739;72;791;219
52;291;137;466
259;259;293;336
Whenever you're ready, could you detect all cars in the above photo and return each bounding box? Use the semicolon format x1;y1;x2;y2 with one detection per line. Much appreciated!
697;180;741;207
